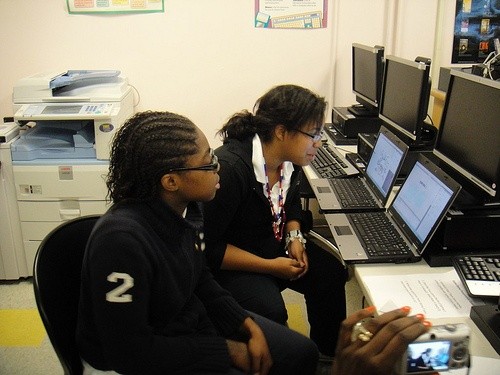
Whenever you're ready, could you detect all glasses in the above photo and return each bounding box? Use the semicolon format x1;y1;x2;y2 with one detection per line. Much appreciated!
167;148;219;174
295;128;323;142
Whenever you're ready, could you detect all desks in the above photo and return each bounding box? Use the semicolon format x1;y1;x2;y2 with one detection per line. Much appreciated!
301;122;500;375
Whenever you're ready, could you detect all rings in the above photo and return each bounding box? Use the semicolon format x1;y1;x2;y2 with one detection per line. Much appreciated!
351;324;373;342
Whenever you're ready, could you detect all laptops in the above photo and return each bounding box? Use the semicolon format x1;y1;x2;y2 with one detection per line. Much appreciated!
309;126;408;212
325;152;462;263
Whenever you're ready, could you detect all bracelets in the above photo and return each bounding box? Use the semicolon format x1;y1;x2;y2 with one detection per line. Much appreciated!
284;230;306;255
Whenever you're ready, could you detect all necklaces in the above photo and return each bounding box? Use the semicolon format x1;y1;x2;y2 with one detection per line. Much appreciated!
265;165;286;241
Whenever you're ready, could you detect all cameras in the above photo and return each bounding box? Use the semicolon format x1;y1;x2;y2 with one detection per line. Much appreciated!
394;322;471;375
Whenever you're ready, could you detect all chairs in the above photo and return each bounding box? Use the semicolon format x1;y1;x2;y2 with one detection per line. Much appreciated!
33;214;106;375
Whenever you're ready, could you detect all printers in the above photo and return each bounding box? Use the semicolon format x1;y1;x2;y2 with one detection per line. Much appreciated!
12;85;137;277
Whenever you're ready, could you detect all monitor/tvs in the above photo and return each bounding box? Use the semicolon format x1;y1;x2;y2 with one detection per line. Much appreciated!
379;55;430;144
433;70;500;197
347;43;382;116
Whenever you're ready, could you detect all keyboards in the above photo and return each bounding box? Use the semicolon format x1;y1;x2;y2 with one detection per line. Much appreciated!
450;255;500;300
309;142;360;180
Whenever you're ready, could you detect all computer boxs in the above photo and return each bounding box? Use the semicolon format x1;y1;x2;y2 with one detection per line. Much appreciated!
358;133;440;184
331;107;382;138
435;199;500;251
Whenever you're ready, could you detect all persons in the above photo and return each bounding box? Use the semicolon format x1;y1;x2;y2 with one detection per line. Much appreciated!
416;348;433;371
80;110;319;375
324;306;432;375
185;84;348;363
